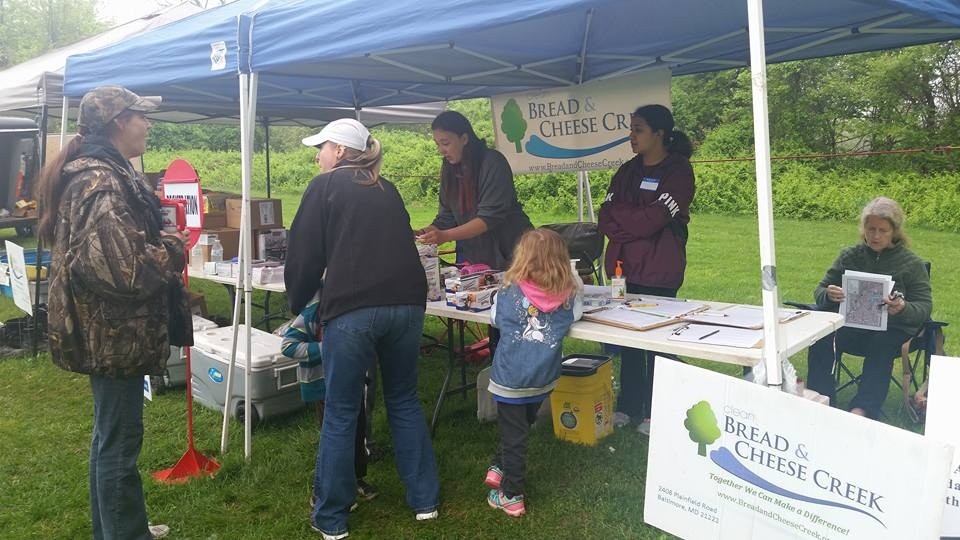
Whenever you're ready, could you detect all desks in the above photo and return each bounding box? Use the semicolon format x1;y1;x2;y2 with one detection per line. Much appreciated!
183;270;290;334
426;269;846;442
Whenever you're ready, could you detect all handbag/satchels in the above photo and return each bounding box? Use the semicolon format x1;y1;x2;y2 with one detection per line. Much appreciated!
908;378;929;423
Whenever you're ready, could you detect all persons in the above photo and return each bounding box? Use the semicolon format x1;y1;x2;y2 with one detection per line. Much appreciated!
281;279;379;514
412;110;534;361
485;227;583;517
35;86;194;540
806;196;932;419
597;105;696;437
284;118;441;540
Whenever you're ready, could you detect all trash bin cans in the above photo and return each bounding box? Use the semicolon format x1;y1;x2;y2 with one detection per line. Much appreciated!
550;353;613;445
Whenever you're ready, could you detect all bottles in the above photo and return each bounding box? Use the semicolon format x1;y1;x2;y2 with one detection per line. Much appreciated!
192;241;203;271
211;240;223;262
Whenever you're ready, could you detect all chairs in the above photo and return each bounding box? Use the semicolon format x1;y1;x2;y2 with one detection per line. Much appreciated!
828;260;949;420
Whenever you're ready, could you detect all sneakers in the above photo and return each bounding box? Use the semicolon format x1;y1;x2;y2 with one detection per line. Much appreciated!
355;479;382;502
487;487;527;518
308;495;361;513
147;524;171;540
414;509;439;521
485;464;504;489
636;418;650;436
307;515;349;540
613;411;632;427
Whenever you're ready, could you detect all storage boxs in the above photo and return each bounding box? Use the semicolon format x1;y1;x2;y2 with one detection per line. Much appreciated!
185;190;290;285
444;276;503;312
0;250;54;306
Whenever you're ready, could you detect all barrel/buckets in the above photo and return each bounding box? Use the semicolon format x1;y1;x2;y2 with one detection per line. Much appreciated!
0;251;52;298
550;354;615;447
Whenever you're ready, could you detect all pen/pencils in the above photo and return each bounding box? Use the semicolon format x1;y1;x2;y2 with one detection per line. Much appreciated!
699;330;719;340
627;304;658;307
672;324;690;335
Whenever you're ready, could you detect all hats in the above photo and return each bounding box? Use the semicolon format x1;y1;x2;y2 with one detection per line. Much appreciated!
77;84;162;136
302;117;372;153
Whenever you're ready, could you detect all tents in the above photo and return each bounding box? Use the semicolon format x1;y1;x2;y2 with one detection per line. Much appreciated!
0;0;960;458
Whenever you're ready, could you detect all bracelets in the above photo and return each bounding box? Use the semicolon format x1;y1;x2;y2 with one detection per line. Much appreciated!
421;229;425;234
